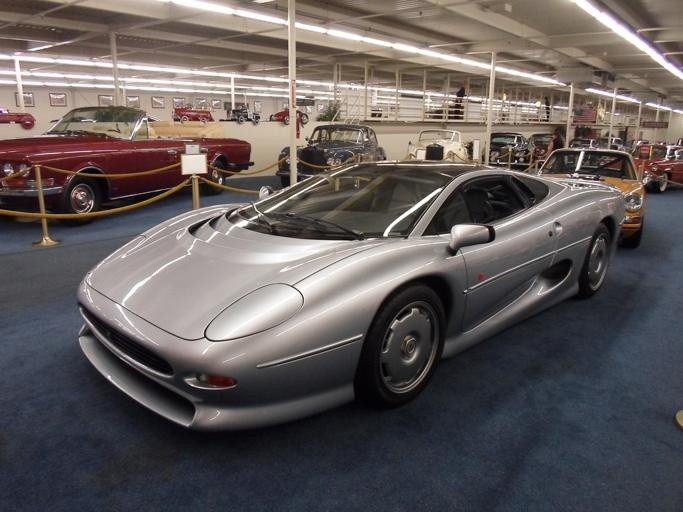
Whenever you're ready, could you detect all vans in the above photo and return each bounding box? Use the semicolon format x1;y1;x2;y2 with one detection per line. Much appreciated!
600;137;623;149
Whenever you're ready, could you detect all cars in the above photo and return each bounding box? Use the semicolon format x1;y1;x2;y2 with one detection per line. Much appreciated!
482;133;531;167
408;130;473;162
536;146;644;250
276;125;386;188
530;133;565;168
569;138;599;149
630;138;683;193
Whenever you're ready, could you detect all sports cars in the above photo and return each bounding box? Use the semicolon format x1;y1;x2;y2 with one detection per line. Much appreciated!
77;162;625;430
0;106;254;225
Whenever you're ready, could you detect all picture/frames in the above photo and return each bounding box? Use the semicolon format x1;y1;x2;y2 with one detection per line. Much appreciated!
253;100;264;113
98;95;115;107
211;99;222;109
172;97;185;108
49;93;69;107
15;92;35;107
193;96;207;110
125;96;141;108
151;96;165;108
306;106;312;114
317;103;324;113
